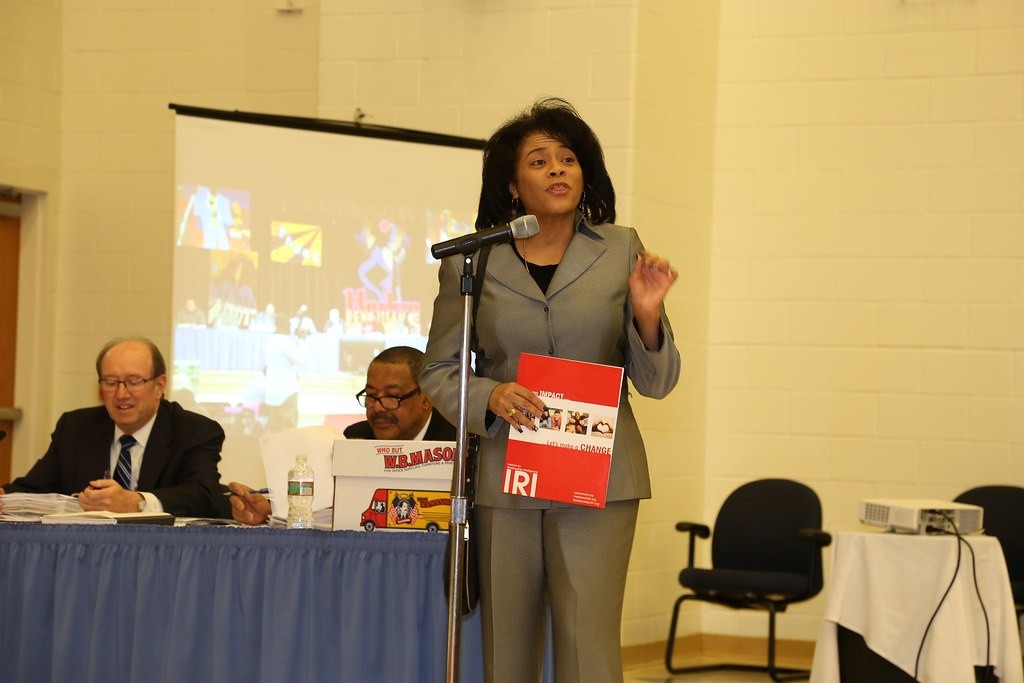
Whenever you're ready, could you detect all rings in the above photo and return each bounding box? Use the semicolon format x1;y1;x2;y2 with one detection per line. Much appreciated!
508;409;516;416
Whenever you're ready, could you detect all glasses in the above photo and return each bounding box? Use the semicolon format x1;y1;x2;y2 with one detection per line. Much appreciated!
356;389;420;409
96;375;159;392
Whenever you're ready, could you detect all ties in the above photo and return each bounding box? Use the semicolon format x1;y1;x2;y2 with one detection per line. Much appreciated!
113;435;137;491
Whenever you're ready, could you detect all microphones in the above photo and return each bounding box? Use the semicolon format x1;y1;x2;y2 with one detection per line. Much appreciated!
431;215;540;260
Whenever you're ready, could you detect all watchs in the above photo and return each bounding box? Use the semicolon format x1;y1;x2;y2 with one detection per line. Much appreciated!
137;493;146;512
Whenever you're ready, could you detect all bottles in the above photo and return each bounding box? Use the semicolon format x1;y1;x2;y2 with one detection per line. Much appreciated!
288;456;314;528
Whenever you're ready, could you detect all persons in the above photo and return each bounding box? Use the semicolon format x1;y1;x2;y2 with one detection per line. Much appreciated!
290;305;316;336
255;304;276;334
324;308;345;334
565;411;588;435
592;420;613;434
229;346;457;526
0;337;225;519
539;410;562;430
418;97;681;683
178;299;206;329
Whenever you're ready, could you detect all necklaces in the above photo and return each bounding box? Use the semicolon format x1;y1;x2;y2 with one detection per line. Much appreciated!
523;239;529;273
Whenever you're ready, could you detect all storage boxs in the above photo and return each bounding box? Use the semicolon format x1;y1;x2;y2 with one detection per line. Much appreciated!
328;437;457;534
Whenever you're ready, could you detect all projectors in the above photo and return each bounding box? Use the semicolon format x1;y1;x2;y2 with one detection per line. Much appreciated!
859;498;984;535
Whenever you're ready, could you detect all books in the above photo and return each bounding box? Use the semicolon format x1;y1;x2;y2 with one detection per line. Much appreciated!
41;512;175;526
501;351;624;508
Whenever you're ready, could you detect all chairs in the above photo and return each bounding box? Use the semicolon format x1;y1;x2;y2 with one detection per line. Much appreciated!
953;484;1024;629
664;478;832;683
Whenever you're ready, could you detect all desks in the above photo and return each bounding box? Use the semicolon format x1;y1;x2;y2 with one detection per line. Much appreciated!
0;518;555;683
808;527;1024;683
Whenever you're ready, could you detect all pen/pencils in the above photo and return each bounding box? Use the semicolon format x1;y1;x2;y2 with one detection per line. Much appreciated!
89;485;95;491
223;487;272;497
